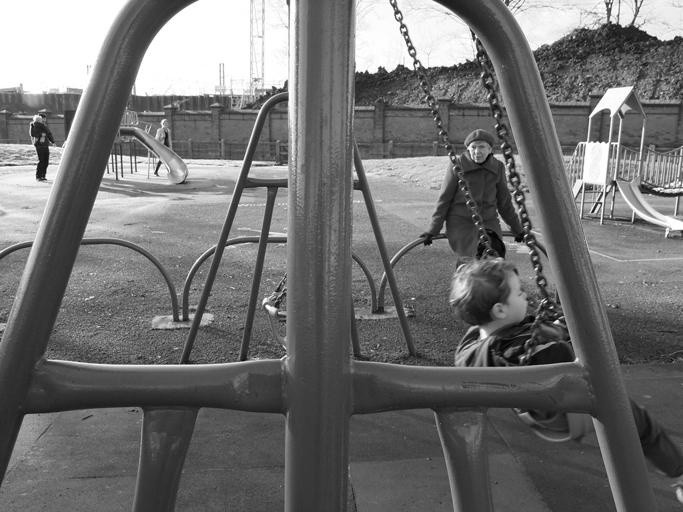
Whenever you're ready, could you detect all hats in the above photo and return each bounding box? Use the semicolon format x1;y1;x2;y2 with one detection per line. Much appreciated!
464;129;496;147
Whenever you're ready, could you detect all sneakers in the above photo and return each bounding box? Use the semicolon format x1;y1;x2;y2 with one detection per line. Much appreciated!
154;172;159;177
37;177;47;181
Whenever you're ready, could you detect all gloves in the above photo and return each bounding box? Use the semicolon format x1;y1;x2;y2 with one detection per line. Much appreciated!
419;232;433;247
514;232;526;245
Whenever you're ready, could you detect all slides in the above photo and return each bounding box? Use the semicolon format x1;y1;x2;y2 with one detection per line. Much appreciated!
616;179;683;231
118;127;187;184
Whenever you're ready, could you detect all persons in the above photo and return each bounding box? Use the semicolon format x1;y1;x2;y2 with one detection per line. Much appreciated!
28;112;57;182
153;118;172;177
448;255;682;507
30;116;46;146
419;128;523;261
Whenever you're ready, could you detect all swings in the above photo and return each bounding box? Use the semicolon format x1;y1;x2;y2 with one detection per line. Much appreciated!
392;1;595;443
